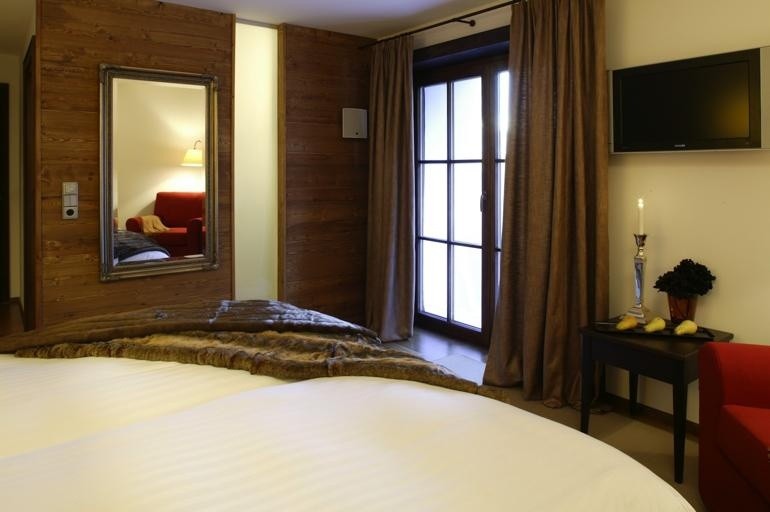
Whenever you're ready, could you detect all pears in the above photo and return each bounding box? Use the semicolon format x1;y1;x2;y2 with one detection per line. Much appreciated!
674;319;698;336
615;315;637;330
643;317;666;332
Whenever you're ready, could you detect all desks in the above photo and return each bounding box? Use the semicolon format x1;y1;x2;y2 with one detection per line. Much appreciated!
572;314;735;483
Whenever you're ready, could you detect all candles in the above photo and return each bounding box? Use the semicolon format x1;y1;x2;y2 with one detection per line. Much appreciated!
636;198;644;237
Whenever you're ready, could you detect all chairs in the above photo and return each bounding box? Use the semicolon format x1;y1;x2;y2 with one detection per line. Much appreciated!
125;191;208;258
697;342;769;512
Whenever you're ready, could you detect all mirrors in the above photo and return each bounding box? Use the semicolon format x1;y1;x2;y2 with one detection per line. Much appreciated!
98;63;220;284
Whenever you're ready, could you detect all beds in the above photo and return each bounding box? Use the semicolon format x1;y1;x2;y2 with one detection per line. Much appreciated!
114;230;171;267
0;298;696;511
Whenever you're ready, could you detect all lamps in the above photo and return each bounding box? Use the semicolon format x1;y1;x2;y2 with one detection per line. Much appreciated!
181;139;205;166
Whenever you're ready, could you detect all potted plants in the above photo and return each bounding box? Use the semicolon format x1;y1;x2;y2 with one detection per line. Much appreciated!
652;259;716;323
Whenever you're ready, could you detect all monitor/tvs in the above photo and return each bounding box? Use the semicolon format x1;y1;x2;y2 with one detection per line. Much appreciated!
611;47;761;151
